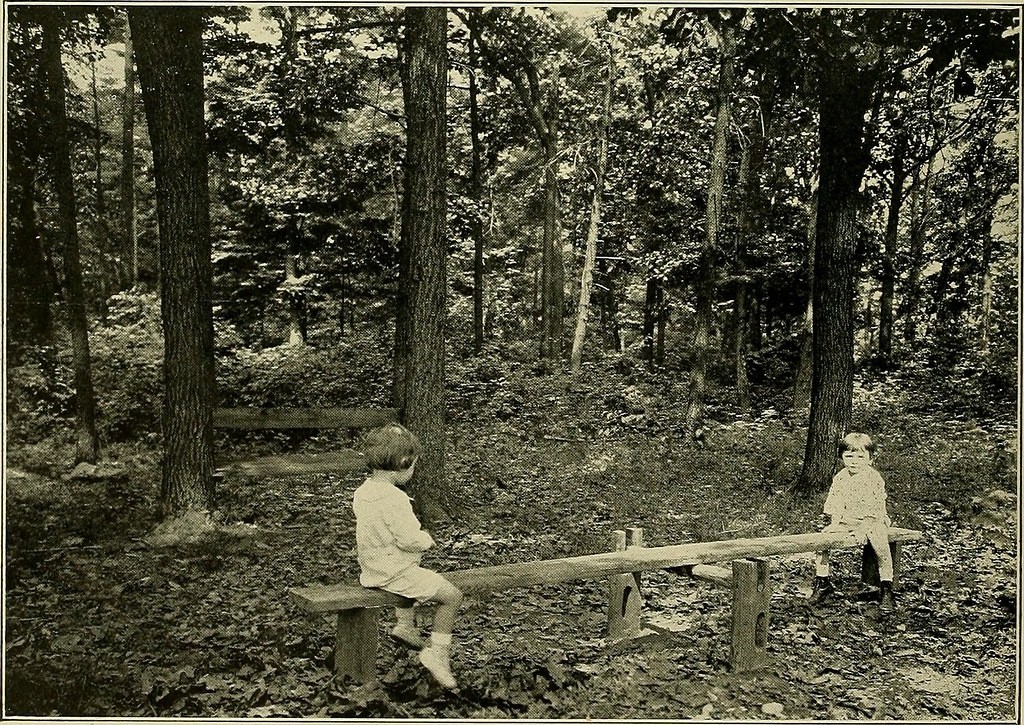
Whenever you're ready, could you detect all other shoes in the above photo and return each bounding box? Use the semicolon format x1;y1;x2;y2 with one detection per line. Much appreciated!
877;580;897;611
806;575;835;606
419;647;458;690
391;627;424;651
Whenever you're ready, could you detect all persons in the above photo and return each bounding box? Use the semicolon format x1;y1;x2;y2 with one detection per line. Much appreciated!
354;420;464;687
808;434;899;614
